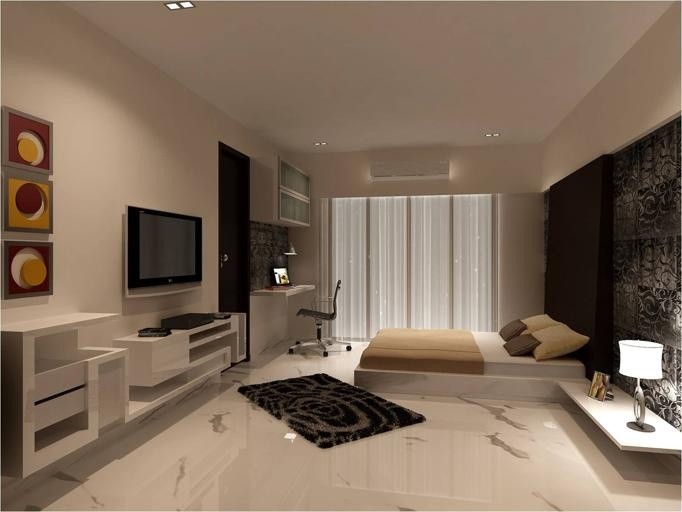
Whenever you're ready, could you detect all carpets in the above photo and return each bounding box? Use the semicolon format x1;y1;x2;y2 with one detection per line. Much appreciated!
238;373;426;449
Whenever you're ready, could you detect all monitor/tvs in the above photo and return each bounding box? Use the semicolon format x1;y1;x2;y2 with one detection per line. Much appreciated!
121;203;203;288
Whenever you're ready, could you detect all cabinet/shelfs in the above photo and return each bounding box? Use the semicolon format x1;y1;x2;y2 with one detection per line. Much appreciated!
1;312;246;479
250;153;311;228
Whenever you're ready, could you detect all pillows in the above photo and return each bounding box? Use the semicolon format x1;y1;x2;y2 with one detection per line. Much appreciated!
499;313;590;361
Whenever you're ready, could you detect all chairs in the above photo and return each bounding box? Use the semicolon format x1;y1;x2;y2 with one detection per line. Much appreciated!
289;280;351;357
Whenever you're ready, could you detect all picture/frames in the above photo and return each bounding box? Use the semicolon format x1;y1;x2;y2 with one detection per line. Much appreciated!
0;106;53;299
588;371;610;402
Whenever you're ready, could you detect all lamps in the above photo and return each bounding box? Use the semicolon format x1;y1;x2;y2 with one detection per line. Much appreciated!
279;242;298;256
618;340;664;432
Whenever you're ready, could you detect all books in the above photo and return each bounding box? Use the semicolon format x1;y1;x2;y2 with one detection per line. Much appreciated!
208;313;231;319
138;328;169;337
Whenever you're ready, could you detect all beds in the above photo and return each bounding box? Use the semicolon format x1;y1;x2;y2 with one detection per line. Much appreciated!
354;328;586;403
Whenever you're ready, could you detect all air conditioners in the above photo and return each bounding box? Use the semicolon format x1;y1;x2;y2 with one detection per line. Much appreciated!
369;159;449;181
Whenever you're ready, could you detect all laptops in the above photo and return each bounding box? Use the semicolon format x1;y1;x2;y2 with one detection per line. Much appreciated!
270;267;294;286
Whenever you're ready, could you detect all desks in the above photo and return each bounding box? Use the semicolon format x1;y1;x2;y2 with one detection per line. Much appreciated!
558;382;682;455
250;284;315;296
